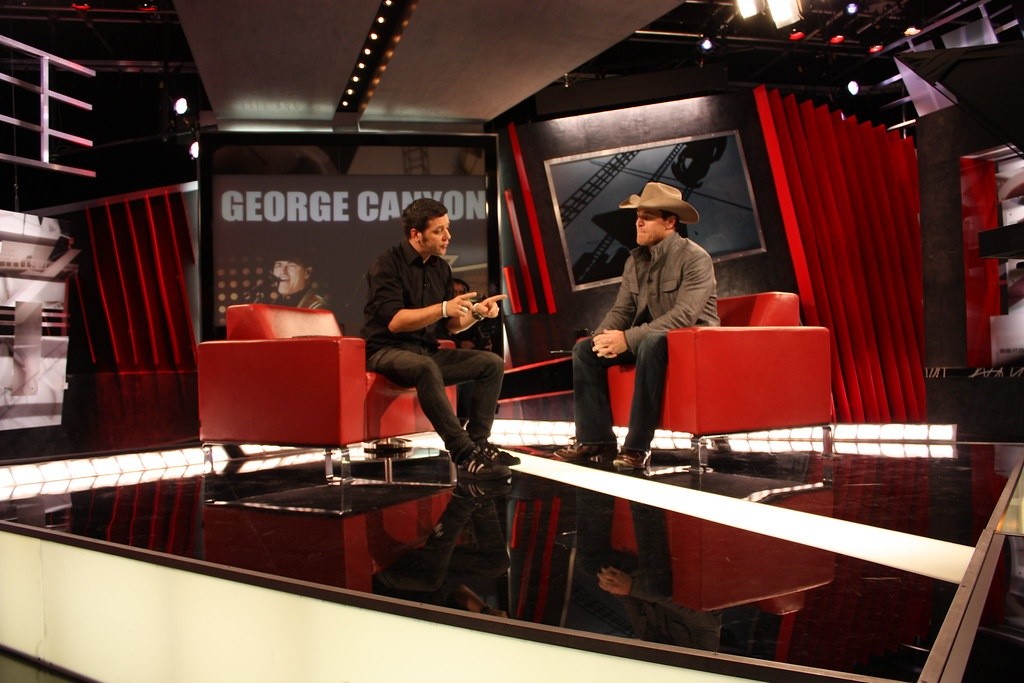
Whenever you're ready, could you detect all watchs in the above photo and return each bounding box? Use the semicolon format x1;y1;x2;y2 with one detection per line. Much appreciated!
472;303;484;320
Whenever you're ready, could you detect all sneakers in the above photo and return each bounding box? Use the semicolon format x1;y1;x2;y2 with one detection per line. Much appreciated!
458;446;512;481
478;441;521;466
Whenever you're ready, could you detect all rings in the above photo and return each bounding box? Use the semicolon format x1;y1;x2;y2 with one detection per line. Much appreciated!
461;307;464;311
497;308;499;312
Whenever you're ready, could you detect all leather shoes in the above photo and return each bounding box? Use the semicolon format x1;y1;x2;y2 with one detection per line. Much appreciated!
554;442;617;461
613;448;651;470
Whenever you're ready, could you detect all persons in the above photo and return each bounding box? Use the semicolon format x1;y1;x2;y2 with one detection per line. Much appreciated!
357;198;520;479
253;242;337;310
553;183;720;475
371;480;510;619
575;489;720;650
452;277;491;352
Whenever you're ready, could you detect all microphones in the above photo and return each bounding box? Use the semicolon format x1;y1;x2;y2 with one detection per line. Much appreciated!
247;275;278;298
647;278;653;283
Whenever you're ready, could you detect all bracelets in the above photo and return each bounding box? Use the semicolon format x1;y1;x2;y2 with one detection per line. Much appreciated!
442;301;448;317
480;605;492;614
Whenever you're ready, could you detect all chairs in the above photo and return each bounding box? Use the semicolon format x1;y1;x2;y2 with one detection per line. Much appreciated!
579;291;835;474
196;303;458;486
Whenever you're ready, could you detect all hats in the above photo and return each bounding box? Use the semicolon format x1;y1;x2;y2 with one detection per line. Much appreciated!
619;182;700;224
